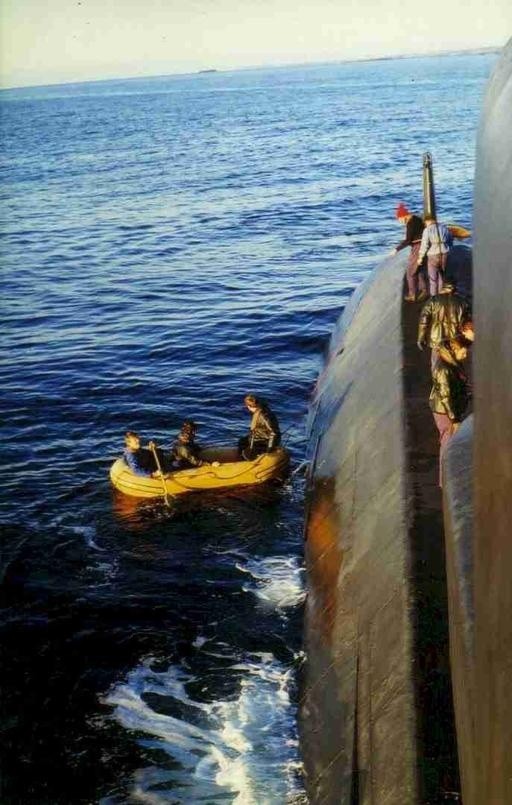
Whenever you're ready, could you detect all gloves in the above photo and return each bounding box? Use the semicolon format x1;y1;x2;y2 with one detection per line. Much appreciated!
389;249;397;256
417;335;426;351
443;403;458;421
417;256;424;266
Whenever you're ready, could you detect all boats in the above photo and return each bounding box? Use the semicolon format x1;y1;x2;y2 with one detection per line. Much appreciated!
109;445;290;500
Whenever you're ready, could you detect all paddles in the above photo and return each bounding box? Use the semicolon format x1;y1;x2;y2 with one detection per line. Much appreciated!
148;439;177;508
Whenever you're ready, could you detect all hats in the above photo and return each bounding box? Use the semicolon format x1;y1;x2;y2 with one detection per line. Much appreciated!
422;213;437;223
395;202;409;217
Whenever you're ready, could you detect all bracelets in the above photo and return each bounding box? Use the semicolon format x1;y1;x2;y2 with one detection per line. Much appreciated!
151;472;154;478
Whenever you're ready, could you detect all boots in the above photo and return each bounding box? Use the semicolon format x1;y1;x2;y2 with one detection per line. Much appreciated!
405;289;428;302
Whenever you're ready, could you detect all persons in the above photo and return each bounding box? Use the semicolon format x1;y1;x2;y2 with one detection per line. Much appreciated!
385;202;429;304
457;314;475;346
170;422;221;472
237;394;280;463
426;332;475;490
415;211;453;298
416;281;469;380
124;431;189;479
